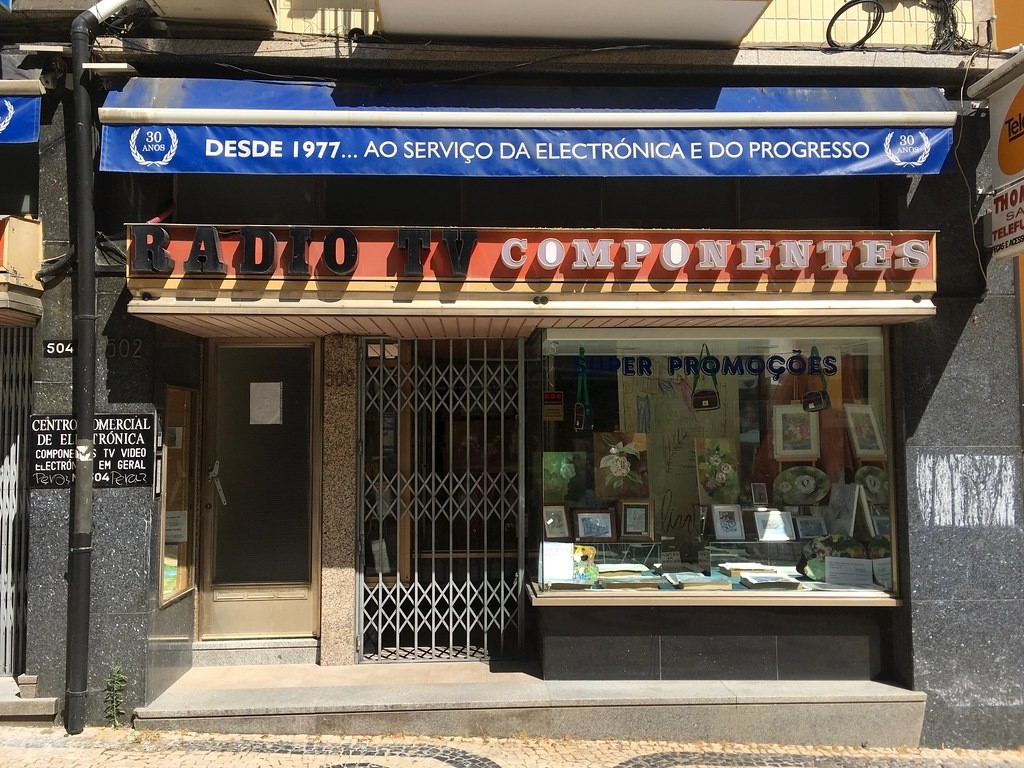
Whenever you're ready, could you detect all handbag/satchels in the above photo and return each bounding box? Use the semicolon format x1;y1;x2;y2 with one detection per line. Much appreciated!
573;403;594;432
692;389;720;411
802;390;830;412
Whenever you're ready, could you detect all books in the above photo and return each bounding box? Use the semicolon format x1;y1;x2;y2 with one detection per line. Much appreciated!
596;561;800;590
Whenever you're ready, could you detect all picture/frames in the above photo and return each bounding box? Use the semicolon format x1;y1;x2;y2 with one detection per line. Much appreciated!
572;509;617;543
541;501;572;541
772;404;821;458
754;512;796;541
796;517;827;538
751;482;768;504
711;504;745;540
844;404;888;458
616;498;654;542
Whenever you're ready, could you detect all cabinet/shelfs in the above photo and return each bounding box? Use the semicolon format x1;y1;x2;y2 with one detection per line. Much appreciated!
525;324;902;682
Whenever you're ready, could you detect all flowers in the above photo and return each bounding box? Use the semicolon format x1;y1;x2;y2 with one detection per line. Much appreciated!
698;441;737;498
599;434;643;493
